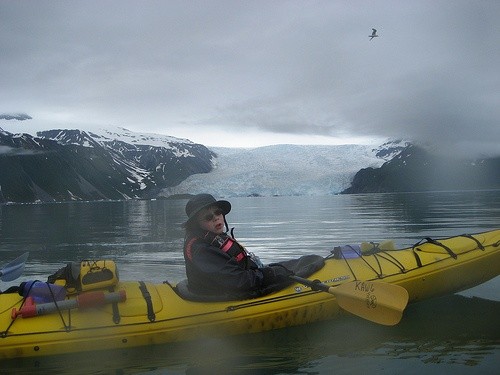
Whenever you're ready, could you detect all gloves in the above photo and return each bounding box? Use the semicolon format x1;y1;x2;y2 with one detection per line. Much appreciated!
272;265;292;281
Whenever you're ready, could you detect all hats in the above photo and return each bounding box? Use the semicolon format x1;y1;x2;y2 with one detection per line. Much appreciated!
182;193;231;228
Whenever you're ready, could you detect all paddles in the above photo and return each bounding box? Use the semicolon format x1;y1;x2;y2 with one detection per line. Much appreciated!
290;274;409;327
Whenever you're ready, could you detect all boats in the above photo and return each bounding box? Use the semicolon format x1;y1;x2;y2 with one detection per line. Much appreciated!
0;228;500;362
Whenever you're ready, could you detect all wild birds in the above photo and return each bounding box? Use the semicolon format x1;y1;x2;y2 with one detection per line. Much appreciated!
368;28;378;40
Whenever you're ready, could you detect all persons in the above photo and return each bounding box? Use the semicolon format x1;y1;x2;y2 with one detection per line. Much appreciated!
180;193;293;295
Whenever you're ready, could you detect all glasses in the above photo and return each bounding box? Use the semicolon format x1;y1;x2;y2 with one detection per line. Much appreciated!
196;208;224;222
